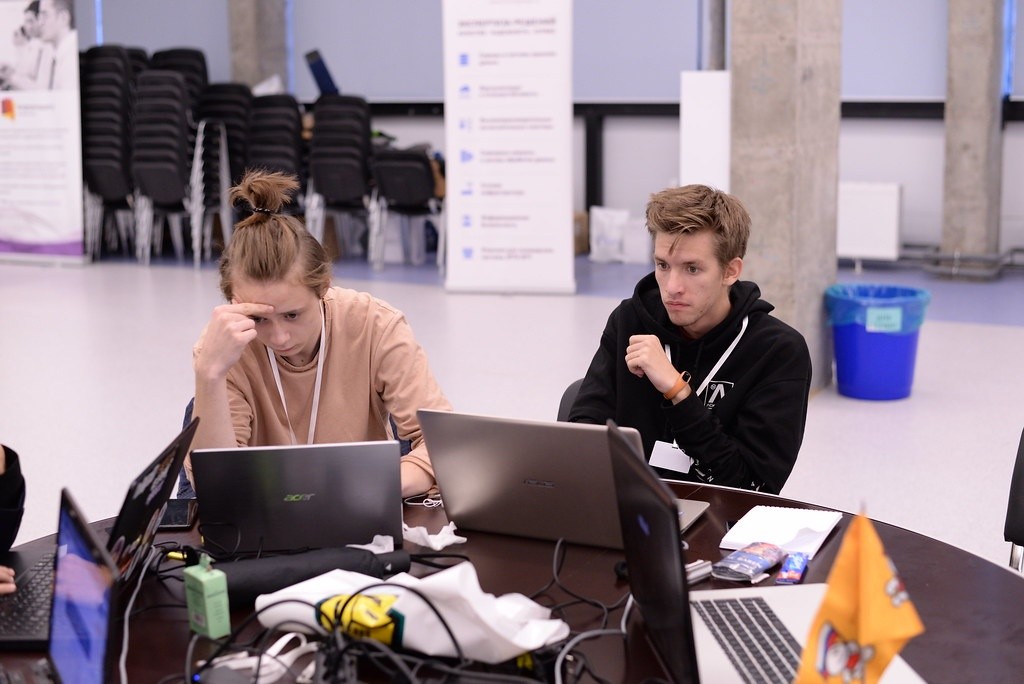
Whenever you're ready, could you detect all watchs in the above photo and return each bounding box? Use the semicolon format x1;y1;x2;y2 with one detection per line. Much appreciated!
664;371;691;400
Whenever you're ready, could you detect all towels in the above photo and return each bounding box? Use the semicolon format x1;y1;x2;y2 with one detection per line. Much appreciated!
254;560;569;663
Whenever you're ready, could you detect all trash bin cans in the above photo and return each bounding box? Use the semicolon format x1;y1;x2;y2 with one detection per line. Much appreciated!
823;283;932;402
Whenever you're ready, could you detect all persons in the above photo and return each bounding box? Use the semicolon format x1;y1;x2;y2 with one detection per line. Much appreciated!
182;171;451;495
0;445;26;596
566;183;813;496
1;0;81;91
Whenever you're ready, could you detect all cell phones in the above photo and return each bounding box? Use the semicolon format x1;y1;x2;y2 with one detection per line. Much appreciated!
156;499;197;531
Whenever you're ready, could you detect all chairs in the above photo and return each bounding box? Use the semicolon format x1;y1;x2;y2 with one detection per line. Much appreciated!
1003;428;1024;572
80;45;447;275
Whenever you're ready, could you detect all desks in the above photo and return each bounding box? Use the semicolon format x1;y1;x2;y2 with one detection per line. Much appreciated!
0;480;1024;684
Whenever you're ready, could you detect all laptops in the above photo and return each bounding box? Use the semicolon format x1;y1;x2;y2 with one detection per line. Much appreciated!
605;422;931;684
0;415;200;646
0;487;120;684
189;440;404;562
414;408;709;548
